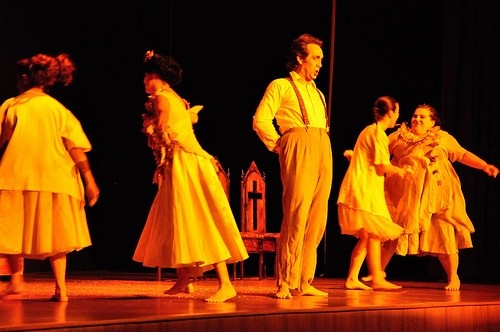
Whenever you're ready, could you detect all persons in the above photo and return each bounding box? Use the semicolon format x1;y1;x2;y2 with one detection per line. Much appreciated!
0;54;99;301
133;50;250;303
337;97;405;290
253;34;333;299
343;104;499;291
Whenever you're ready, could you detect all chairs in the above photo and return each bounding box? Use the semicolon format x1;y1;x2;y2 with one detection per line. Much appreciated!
156;157;279;283
